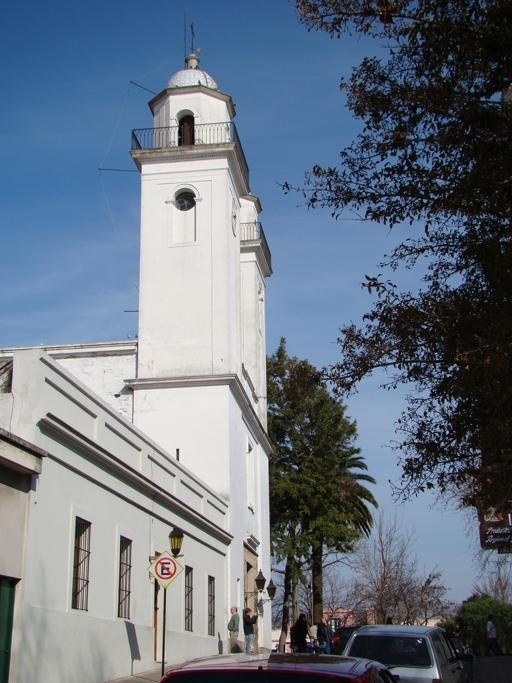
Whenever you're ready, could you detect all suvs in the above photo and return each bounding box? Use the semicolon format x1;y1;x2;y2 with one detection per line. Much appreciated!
306;623;474;682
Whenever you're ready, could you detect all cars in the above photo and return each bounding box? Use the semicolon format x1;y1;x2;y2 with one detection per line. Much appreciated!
158;651;401;682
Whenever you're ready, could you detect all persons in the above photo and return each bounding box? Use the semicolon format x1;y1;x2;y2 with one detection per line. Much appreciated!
316;615;328;653
226;604;238;652
289;612;310;653
243;606;257;654
483;612;502;658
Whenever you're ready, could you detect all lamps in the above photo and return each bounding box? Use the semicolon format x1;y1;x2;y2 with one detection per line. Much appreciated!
254;568;276;599
168;526;183;557
